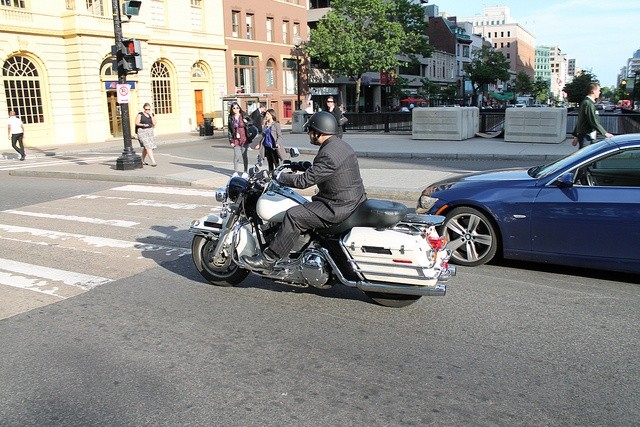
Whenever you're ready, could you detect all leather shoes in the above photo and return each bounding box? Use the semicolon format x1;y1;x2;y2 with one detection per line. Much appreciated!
241;252;277;275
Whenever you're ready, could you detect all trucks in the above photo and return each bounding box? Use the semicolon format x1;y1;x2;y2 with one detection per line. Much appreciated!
514;96;534;107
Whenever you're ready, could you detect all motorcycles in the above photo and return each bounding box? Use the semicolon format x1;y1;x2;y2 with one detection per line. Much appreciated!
187;146;457;308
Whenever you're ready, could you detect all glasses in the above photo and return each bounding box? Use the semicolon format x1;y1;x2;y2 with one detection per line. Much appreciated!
326;100;334;104
232;106;240;110
145;108;151;111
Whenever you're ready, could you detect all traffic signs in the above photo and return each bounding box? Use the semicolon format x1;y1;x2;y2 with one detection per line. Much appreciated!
115;83;130;104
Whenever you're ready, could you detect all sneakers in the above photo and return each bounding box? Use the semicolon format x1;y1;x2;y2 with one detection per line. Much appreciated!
232;172;239;178
241;172;248;179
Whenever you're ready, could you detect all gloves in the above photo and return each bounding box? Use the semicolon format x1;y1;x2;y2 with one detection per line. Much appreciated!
297;160;311;172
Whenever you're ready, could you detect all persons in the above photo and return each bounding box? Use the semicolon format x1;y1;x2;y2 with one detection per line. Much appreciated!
135;102;157;166
227;102;257;179
572;83;613;150
254;109;286;170
247;104;266;150
8;111;26;161
326;96;344;139
239;112;367;274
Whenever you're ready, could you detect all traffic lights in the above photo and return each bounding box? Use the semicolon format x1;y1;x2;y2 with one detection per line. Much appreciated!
121;40;142;75
123;1;141;17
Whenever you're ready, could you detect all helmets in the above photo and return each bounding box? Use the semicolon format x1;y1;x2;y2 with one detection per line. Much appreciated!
303;110;339;136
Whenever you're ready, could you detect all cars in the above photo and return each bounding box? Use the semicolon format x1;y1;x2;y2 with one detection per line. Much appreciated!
604;104;615;112
415;131;640;277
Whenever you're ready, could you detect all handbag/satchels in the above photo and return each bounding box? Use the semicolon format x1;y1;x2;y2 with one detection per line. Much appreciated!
243;113;257;140
337;112;348;126
